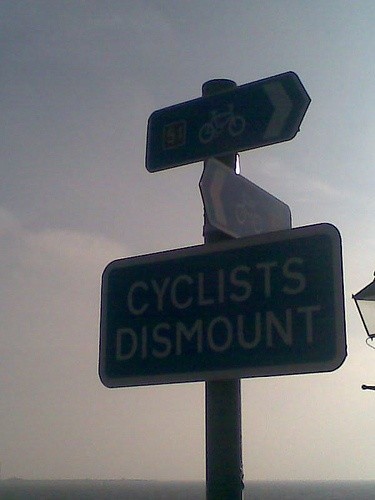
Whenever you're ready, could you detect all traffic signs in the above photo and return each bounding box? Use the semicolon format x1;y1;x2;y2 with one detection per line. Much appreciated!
145;70;313;173
198;158;292;239
